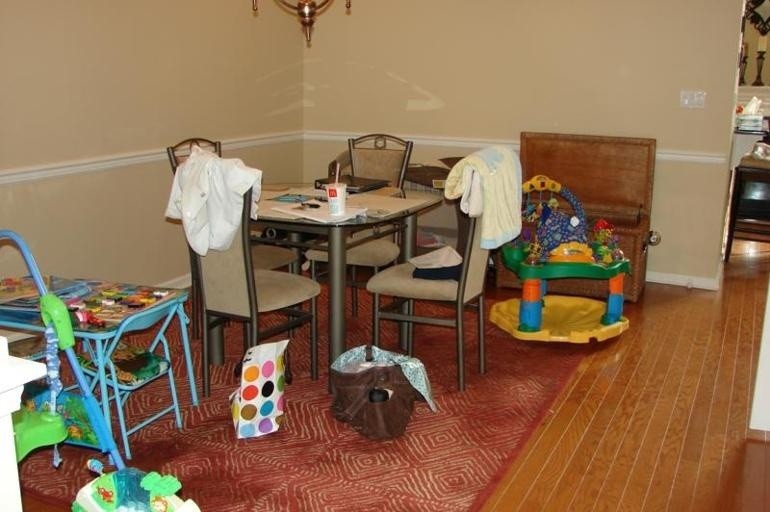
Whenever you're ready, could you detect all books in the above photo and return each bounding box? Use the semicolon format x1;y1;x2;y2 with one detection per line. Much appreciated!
315;174;391;194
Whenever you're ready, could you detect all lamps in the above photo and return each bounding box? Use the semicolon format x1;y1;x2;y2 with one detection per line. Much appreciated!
252;0;351;43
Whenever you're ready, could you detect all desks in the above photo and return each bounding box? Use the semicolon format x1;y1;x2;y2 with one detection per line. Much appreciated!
0;274;203;466
724;154;770;262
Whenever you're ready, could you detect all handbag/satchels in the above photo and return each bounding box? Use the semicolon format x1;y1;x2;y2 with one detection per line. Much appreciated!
228;339;291;441
328;342;426;442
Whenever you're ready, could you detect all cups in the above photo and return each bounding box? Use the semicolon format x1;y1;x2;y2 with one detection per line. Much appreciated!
326;182;347;217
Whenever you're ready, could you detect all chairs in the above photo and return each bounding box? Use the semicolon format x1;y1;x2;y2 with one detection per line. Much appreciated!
172;152;322;399
366;144;524;391
304;132;413;321
80;298;183;462
167;137;299;339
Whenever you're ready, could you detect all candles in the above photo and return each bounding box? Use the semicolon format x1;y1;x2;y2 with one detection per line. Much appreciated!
758;35;767;51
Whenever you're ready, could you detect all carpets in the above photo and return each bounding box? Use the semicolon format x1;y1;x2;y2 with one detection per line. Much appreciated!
17;268;590;512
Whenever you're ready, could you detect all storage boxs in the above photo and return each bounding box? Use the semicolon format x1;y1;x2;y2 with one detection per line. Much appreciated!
497;132;656;304
